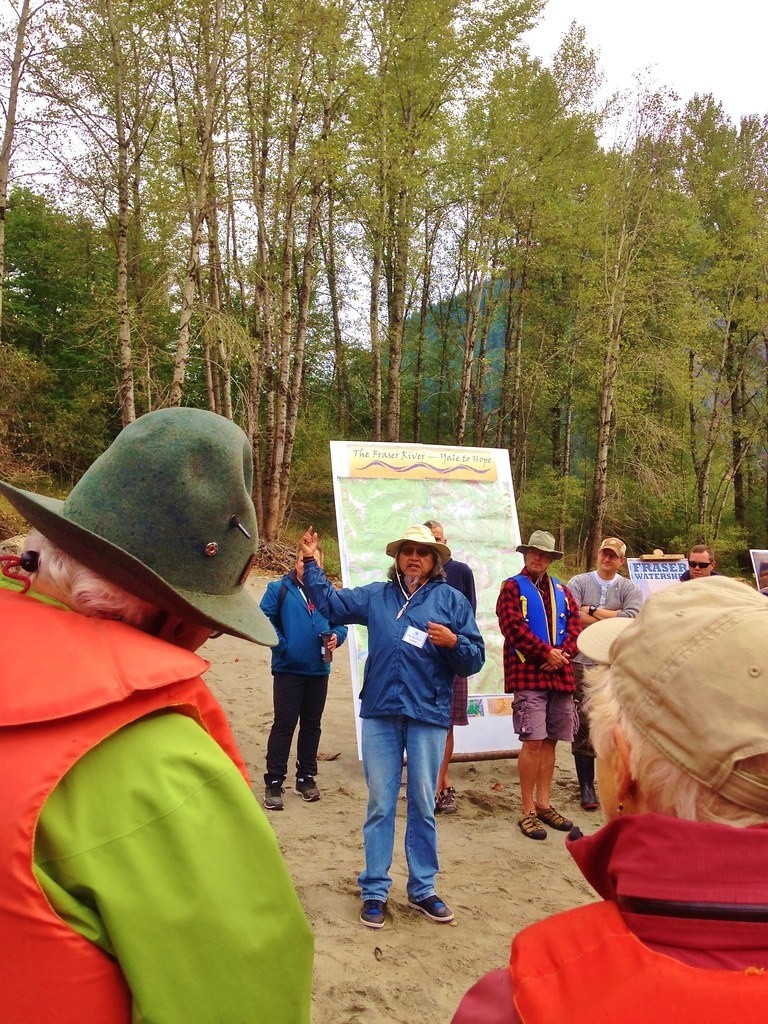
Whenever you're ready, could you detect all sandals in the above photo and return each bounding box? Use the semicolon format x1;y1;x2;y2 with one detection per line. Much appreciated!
535;803;573;831
518;810;547;840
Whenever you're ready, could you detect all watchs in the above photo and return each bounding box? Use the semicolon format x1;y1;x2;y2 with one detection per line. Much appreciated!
588;604;597;616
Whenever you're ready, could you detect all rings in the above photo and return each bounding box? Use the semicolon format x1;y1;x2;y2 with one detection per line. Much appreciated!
429;635;434;639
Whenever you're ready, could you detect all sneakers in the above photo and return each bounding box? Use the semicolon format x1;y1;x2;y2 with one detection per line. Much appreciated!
263;773;286;810
408;895;454;921
294;773;320;802
360;898;387;927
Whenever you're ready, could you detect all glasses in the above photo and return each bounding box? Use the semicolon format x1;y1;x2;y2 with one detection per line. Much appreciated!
210;626;224;639
397;547;438;558
688;560;712;569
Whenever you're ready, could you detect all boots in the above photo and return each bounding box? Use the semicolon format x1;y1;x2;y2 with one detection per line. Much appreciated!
576;756;601;810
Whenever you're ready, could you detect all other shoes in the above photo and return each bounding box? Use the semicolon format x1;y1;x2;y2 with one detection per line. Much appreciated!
439;785;457;813
434;794;440;816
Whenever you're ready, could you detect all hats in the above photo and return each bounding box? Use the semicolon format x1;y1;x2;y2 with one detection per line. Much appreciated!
385;525;451;565
576;571;768;814
515;530;564;561
598;537;627;558
0;406;279;649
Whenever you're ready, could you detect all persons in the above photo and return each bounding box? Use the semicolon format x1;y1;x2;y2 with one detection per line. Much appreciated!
759;563;768;589
449;573;768;1024
0;405;315;1024
294;520;486;930
256;536;348;811
493;531;586;841
680;544;719;582
566;533;642;809
